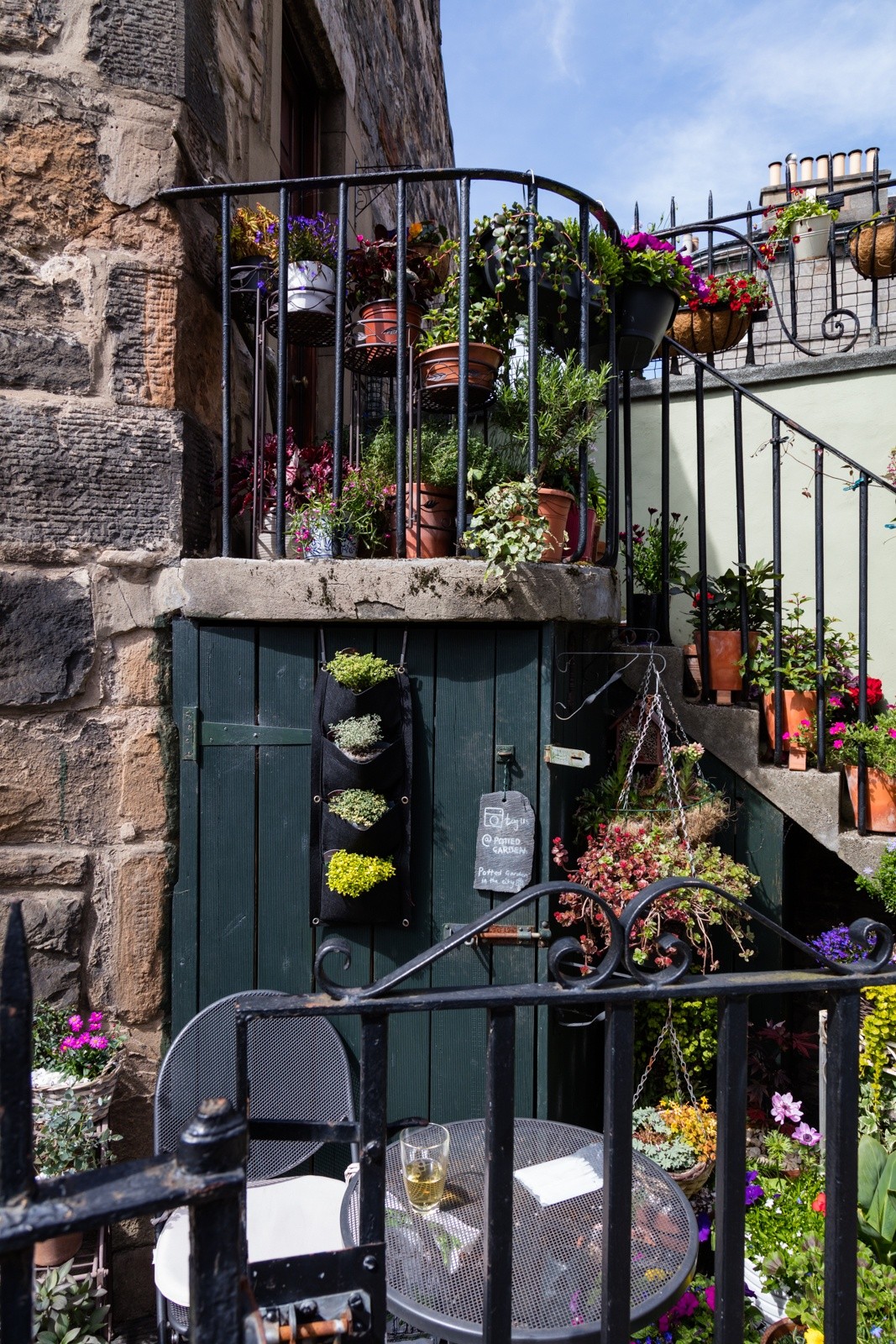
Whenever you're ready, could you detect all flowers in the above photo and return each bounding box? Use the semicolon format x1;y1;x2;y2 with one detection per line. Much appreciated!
1;187;896;1344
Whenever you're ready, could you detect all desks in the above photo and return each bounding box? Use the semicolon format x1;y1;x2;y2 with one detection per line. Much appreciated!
342;1115;701;1344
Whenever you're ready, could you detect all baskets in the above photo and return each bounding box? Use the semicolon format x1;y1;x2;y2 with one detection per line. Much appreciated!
32;1044;129;1123
666;1157;716;1200
392;219;450;285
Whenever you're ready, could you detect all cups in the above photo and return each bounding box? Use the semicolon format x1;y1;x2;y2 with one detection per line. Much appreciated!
399;1122;450;1215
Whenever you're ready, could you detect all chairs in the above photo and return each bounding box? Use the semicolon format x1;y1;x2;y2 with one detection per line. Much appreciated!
151;992;386;1344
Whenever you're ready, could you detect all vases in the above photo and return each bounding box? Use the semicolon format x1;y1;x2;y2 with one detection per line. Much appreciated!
298;506;359;559
786;214;832;262
252;506;297;560
630;593;666;643
608;283;683;371
283;262;335;311
844;762;895;835
31;1230;84;1266
693;629;761;690
762;687;817;752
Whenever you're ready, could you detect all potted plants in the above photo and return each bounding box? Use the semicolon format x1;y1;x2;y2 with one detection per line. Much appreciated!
546;458;602;562
381;428;478;559
482;351;612;563
419;299;520;403
476;211;625;328
